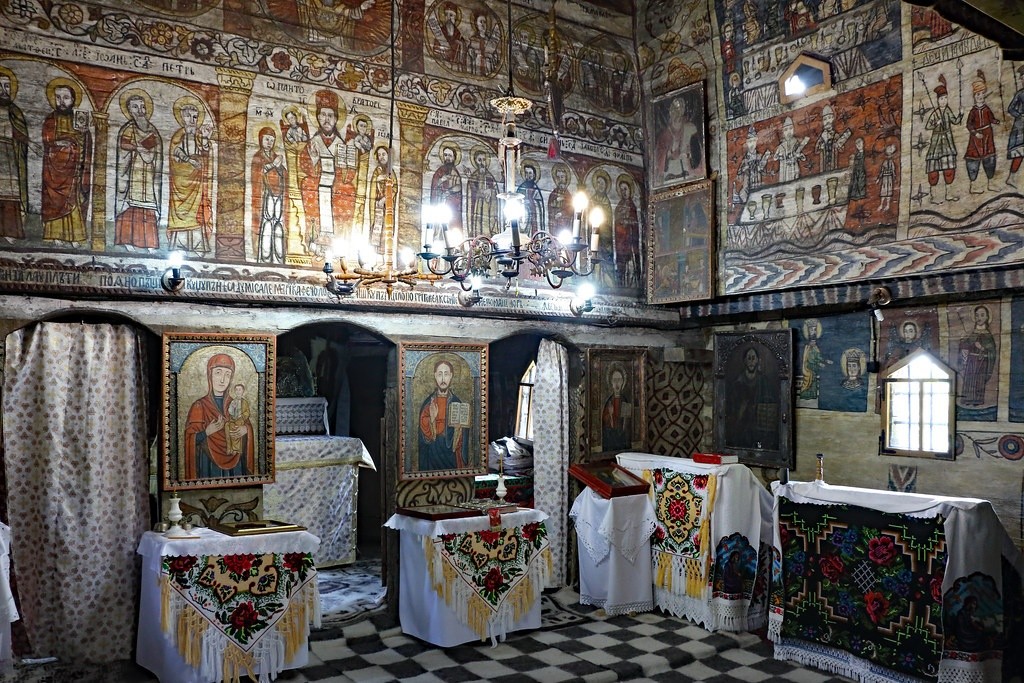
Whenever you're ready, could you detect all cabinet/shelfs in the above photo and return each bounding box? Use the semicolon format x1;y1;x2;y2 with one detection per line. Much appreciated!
568;486;659;617
475;472;535;510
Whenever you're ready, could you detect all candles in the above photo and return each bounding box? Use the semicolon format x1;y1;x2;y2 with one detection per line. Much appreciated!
173;482;176;493
498;452;504;473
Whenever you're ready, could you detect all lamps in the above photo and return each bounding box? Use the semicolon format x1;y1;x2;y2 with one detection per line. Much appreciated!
866;287;892;323
161;250;186;293
414;126;608;292
321;0;443;300
488;0;534;150
570;283;596;316
458;275;483;308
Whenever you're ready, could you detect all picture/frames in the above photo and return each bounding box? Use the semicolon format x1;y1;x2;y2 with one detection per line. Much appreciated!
398;340;489;483
586;347;646;463
161;331;276;492
646;179;717;306
651;79;709;194
706;327;796;472
